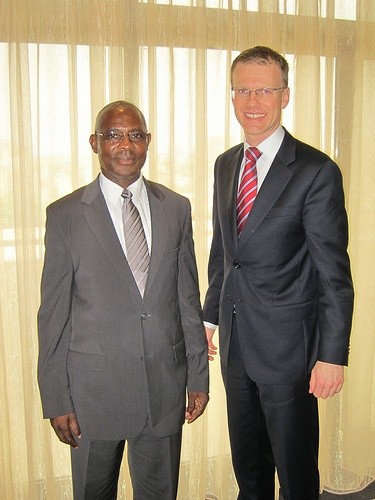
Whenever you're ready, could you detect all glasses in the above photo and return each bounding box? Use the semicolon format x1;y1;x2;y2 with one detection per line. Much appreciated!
230;86;282;100
95;129;146;143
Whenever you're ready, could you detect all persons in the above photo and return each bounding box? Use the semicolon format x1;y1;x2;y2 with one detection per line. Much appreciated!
37;100;209;500
201;46;355;500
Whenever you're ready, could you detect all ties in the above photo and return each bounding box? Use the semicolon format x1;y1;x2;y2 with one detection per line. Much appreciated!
121;188;150;294
236;147;261;242
